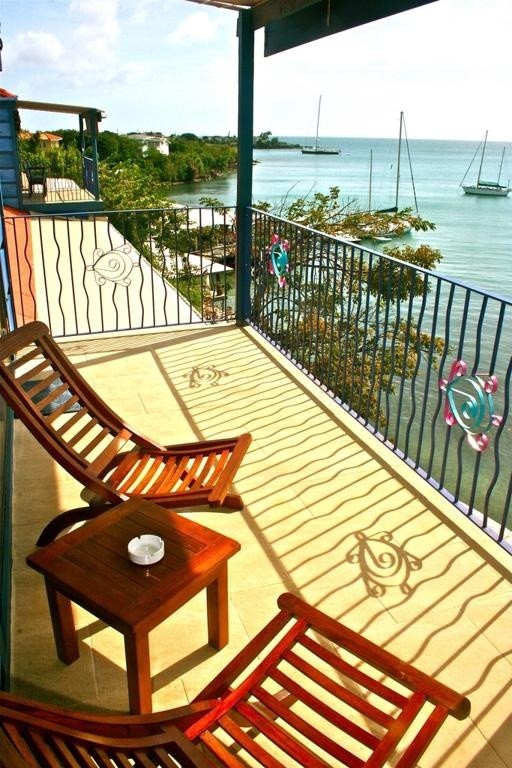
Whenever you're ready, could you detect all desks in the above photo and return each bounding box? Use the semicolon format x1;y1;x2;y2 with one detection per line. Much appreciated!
26;495;242;714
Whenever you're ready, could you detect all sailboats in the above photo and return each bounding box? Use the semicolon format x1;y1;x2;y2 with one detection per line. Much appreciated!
459;129;511;196
294;111;420;242
302;95;342;155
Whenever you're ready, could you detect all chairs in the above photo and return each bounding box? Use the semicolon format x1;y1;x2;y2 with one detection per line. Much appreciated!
23;159;47;198
0;592;471;768
0;320;252;547
